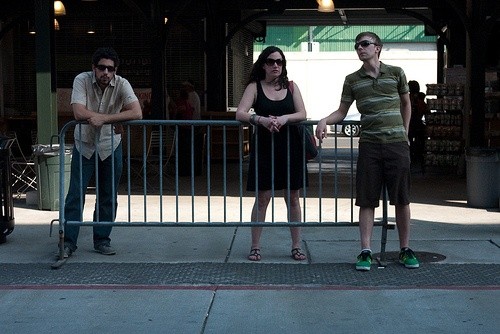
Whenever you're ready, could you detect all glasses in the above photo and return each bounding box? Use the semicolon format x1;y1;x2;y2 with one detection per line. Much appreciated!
264;58;283;66
354;40;377;50
94;65;116;72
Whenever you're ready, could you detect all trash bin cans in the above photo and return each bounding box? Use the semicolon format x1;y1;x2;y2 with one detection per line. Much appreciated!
463;146;500;208
30;144;73;211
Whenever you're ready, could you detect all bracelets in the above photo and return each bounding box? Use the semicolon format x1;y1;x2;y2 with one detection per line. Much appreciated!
258;116;261;124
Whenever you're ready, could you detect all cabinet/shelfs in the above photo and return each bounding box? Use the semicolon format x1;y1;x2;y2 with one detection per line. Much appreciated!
424;84;500;168
208;111;250;160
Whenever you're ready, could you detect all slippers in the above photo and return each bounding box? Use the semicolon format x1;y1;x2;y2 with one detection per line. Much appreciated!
291;248;306;261
249;249;261;261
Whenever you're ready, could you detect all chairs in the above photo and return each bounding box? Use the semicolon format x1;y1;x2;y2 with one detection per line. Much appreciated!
133;130;175;191
0;128;40;199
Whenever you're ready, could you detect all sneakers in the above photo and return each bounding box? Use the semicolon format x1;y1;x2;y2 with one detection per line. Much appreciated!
94;243;116;255
398;248;419;268
55;245;70;258
356;250;373;270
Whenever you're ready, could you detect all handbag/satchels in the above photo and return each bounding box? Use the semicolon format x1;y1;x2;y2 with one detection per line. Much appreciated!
299;124;319;160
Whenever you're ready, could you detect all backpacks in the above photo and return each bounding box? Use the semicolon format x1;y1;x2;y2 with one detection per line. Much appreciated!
168;86;194;130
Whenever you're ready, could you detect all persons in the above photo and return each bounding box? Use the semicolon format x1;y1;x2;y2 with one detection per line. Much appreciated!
316;33;420;271
236;46;310;260
55;50;142;255
168;81;203;176
407;81;433;174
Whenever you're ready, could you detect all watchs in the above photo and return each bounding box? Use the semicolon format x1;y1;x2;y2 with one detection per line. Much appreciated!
250;114;257;125
